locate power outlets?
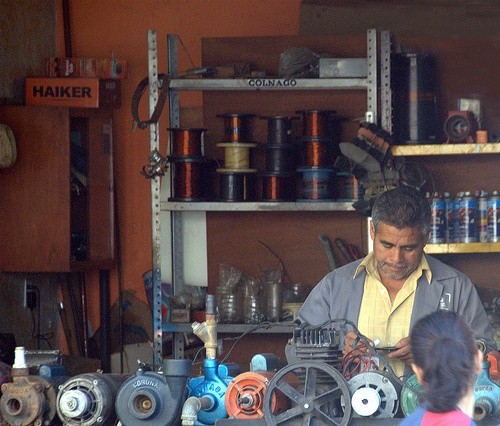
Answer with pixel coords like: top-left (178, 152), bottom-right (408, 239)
top-left (24, 279), bottom-right (37, 308)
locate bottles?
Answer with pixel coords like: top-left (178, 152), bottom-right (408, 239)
top-left (426, 191), bottom-right (500, 243)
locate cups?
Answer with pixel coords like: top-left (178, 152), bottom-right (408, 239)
top-left (260, 283), bottom-right (283, 322)
top-left (214, 285), bottom-right (242, 321)
top-left (242, 295), bottom-right (262, 323)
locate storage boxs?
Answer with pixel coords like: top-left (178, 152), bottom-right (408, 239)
top-left (25, 77), bottom-right (118, 109)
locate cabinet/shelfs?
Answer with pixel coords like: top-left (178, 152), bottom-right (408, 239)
top-left (149, 27), bottom-right (379, 371)
top-left (380, 30), bottom-right (500, 254)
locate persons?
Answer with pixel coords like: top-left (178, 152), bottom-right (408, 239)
top-left (285, 187), bottom-right (498, 418)
top-left (395, 310), bottom-right (483, 426)
